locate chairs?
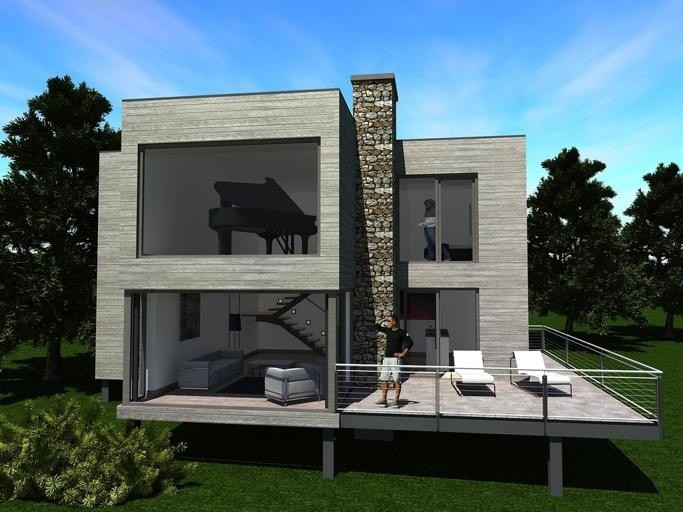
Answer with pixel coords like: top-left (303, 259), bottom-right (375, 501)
top-left (450, 348), bottom-right (498, 398)
top-left (507, 349), bottom-right (574, 397)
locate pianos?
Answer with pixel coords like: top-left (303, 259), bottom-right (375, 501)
top-left (207, 178), bottom-right (319, 253)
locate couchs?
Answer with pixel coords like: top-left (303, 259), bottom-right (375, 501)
top-left (264, 363), bottom-right (323, 407)
top-left (175, 350), bottom-right (247, 390)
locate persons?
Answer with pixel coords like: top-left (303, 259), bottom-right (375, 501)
top-left (415, 198), bottom-right (445, 262)
top-left (374, 315), bottom-right (414, 409)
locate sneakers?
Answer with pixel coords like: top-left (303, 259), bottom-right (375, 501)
top-left (392, 400), bottom-right (399, 408)
top-left (376, 400), bottom-right (387, 407)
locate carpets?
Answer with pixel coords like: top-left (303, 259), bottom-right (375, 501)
top-left (214, 376), bottom-right (268, 395)
